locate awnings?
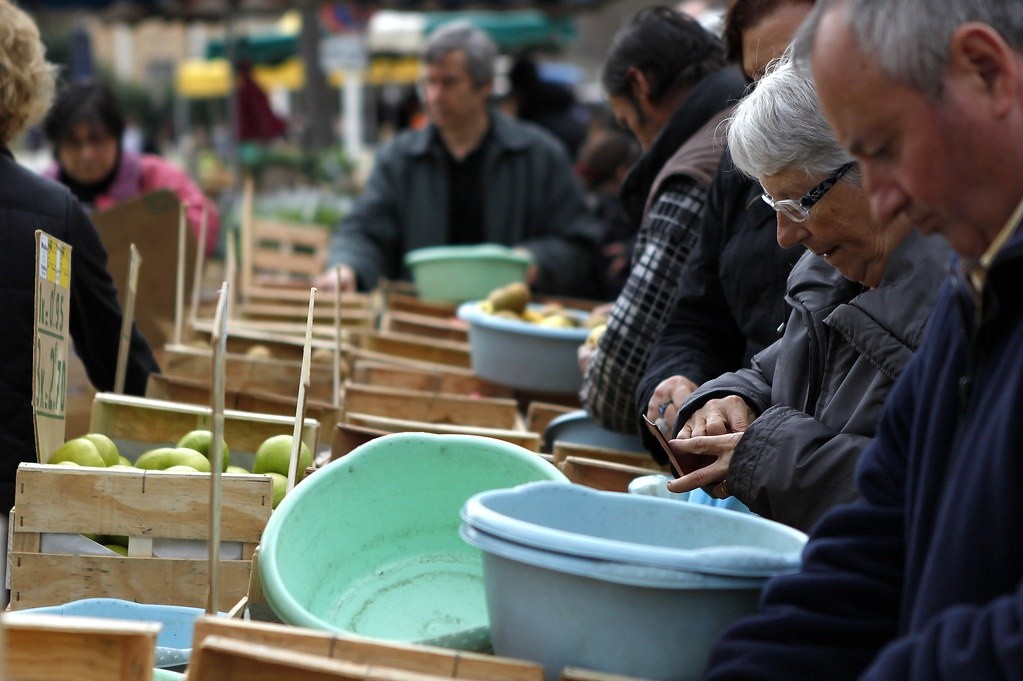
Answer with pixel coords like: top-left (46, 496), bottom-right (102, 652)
top-left (367, 11), bottom-right (575, 48)
top-left (207, 33), bottom-right (327, 63)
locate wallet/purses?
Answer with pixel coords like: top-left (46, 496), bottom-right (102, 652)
top-left (641, 411), bottom-right (740, 491)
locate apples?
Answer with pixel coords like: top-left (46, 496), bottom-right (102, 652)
top-left (48, 430), bottom-right (312, 557)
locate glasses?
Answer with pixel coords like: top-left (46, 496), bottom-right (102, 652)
top-left (762, 162), bottom-right (854, 222)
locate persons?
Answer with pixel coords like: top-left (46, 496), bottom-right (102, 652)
top-left (234, 60), bottom-right (290, 144)
top-left (316, 0), bottom-right (752, 303)
top-left (666, 60), bottom-right (954, 535)
top-left (39, 81), bottom-right (219, 253)
top-left (0, 0), bottom-right (161, 615)
top-left (633, 0), bottom-right (816, 470)
top-left (701, 0), bottom-right (1023, 681)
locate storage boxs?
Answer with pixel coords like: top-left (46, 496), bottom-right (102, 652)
top-left (0, 275), bottom-right (711, 681)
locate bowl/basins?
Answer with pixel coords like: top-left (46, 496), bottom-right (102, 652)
top-left (628, 474), bottom-right (764, 520)
top-left (258, 430), bottom-right (572, 657)
top-left (457, 482), bottom-right (815, 679)
top-left (541, 409), bottom-right (652, 454)
top-left (6, 598), bottom-right (231, 669)
top-left (456, 295), bottom-right (606, 394)
top-left (404, 243), bottom-right (532, 304)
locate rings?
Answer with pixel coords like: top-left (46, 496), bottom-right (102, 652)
top-left (721, 480), bottom-right (730, 497)
top-left (655, 418), bottom-right (670, 435)
top-left (659, 400), bottom-right (673, 418)
